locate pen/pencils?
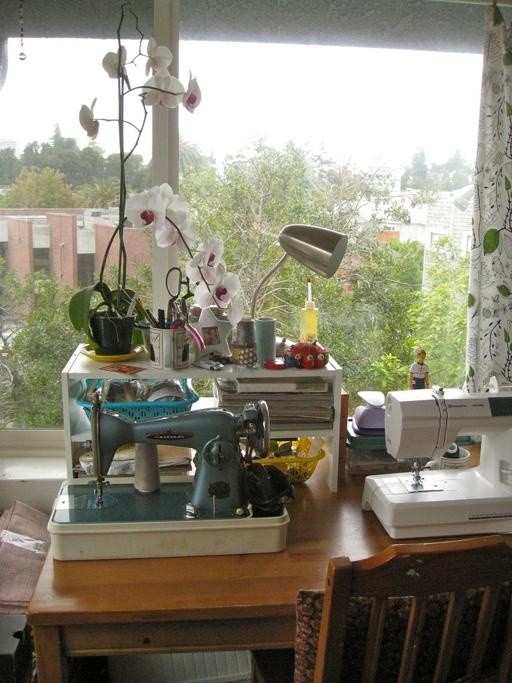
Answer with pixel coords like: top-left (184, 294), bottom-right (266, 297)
top-left (147, 309), bottom-right (165, 329)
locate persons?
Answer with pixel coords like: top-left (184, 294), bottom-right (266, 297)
top-left (408, 348), bottom-right (430, 389)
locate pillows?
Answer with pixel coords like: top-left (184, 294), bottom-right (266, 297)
top-left (293, 583), bottom-right (512, 683)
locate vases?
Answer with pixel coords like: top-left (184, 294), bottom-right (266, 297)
top-left (91, 313), bottom-right (134, 355)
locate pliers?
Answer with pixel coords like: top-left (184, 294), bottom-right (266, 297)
top-left (171, 299), bottom-right (205, 353)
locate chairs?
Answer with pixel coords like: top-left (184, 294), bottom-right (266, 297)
top-left (249, 533), bottom-right (512, 683)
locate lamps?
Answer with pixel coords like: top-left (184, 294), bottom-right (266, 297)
top-left (250, 223), bottom-right (349, 318)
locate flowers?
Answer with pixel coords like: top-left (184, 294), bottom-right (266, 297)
top-left (69, 2), bottom-right (246, 350)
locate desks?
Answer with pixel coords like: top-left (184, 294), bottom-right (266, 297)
top-left (27, 440), bottom-right (512, 683)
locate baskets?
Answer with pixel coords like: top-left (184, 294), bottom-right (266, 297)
top-left (75, 379), bottom-right (199, 421)
top-left (243, 441), bottom-right (326, 482)
